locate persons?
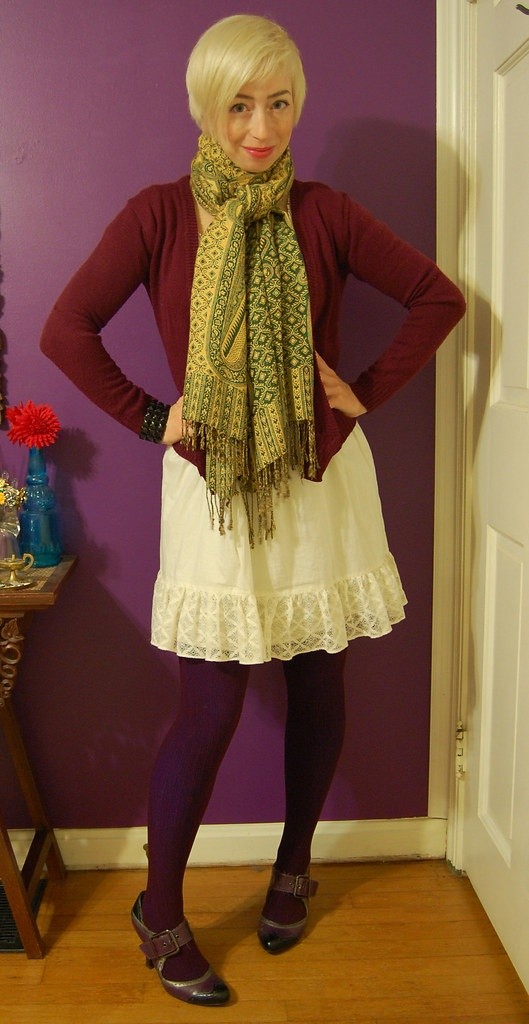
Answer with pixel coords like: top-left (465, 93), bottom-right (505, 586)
top-left (39, 13), bottom-right (470, 1006)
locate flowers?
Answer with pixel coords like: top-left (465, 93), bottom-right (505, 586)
top-left (4, 400), bottom-right (63, 449)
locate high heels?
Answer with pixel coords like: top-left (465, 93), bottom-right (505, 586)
top-left (258, 861), bottom-right (319, 955)
top-left (131, 890), bottom-right (230, 1005)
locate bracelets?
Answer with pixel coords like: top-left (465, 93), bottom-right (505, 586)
top-left (140, 397), bottom-right (169, 444)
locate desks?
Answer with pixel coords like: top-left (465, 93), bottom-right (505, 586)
top-left (0, 553), bottom-right (80, 958)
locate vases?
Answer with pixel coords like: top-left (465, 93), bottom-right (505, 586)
top-left (18, 447), bottom-right (64, 569)
